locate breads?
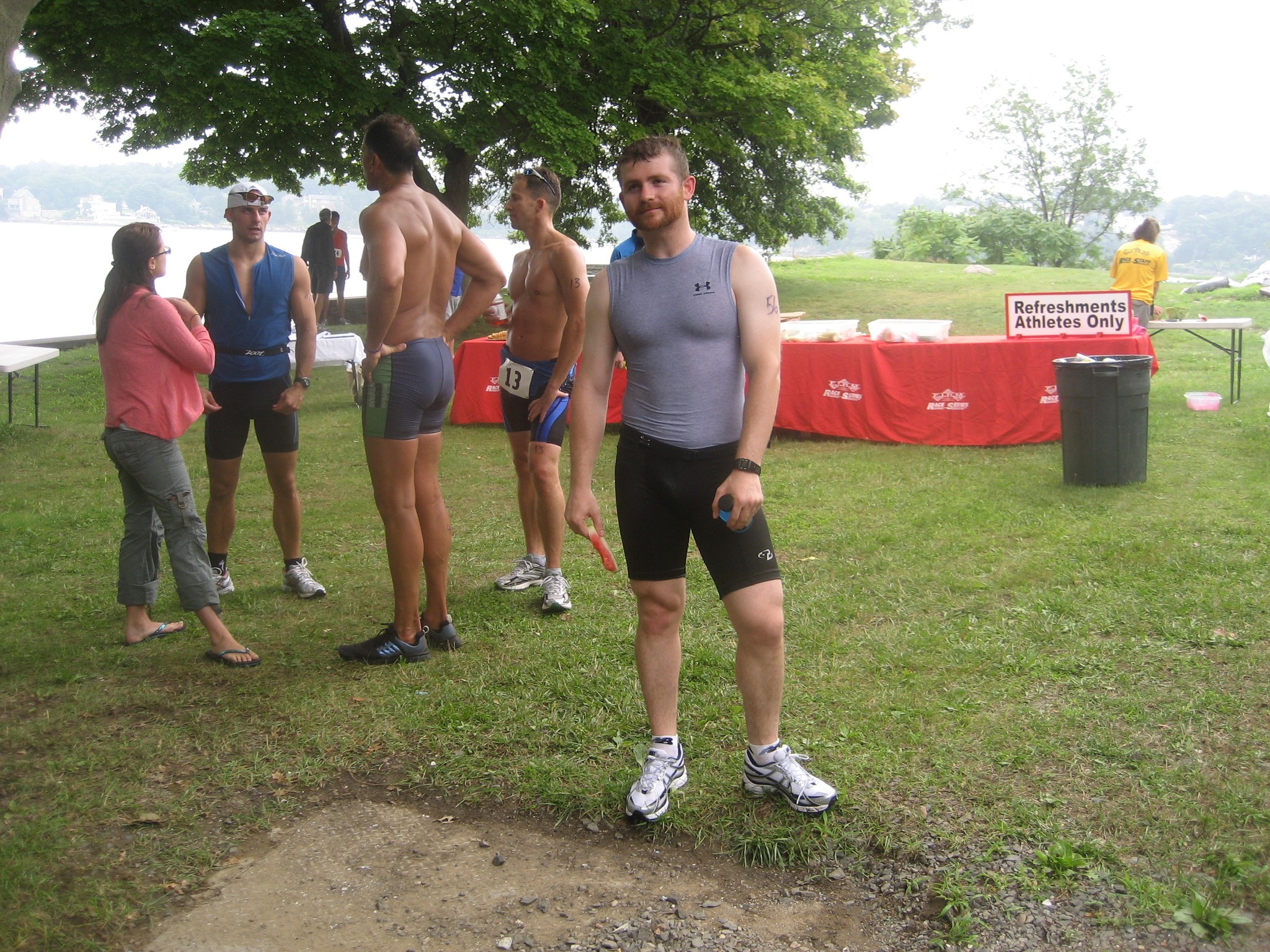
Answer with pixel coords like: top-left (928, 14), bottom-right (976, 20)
top-left (487, 330), bottom-right (507, 339)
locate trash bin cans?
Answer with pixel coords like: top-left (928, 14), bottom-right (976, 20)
top-left (1051, 354), bottom-right (1152, 487)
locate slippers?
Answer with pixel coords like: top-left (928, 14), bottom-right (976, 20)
top-left (125, 622), bottom-right (186, 646)
top-left (205, 647), bottom-right (261, 668)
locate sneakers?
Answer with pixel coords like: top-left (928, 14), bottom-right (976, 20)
top-left (211, 567), bottom-right (235, 595)
top-left (338, 621), bottom-right (430, 665)
top-left (281, 557), bottom-right (326, 598)
top-left (539, 573), bottom-right (572, 611)
top-left (742, 743), bottom-right (838, 812)
top-left (419, 611), bottom-right (462, 651)
top-left (626, 742), bottom-right (688, 821)
top-left (496, 554), bottom-right (546, 590)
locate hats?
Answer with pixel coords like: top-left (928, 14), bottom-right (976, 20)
top-left (227, 182), bottom-right (270, 208)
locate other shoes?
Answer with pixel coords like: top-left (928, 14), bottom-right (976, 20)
top-left (316, 323), bottom-right (329, 332)
top-left (337, 317), bottom-right (351, 325)
top-left (320, 318), bottom-right (329, 326)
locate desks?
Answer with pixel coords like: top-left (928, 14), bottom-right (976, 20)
top-left (0, 343), bottom-right (60, 430)
top-left (451, 327), bottom-right (1151, 448)
top-left (286, 333), bottom-right (361, 410)
top-left (1151, 317), bottom-right (1252, 404)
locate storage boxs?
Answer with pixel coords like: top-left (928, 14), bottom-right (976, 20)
top-left (781, 319), bottom-right (860, 343)
top-left (867, 319), bottom-right (952, 342)
top-left (1183, 391), bottom-right (1222, 411)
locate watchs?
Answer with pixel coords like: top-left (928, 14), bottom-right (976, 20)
top-left (294, 377), bottom-right (311, 387)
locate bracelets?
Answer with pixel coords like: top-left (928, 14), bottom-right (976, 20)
top-left (734, 458), bottom-right (761, 476)
top-left (364, 342), bottom-right (383, 354)
top-left (190, 314), bottom-right (200, 321)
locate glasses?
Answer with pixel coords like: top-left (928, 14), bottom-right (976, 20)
top-left (145, 246), bottom-right (171, 264)
top-left (228, 192), bottom-right (275, 205)
top-left (523, 167), bottom-right (557, 196)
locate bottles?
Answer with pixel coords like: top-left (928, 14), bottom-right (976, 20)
top-left (718, 495), bottom-right (754, 533)
top-left (487, 293), bottom-right (509, 326)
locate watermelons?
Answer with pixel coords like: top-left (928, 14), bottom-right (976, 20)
top-left (1166, 306), bottom-right (1192, 321)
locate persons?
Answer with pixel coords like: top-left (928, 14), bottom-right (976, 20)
top-left (182, 182), bottom-right (326, 599)
top-left (609, 228), bottom-right (647, 369)
top-left (96, 222), bottom-right (258, 667)
top-left (1111, 218), bottom-right (1168, 332)
top-left (483, 166), bottom-right (590, 613)
top-left (446, 266), bottom-right (465, 361)
top-left (337, 114), bottom-right (506, 665)
top-left (301, 208), bottom-right (350, 333)
top-left (564, 135), bottom-right (839, 825)
